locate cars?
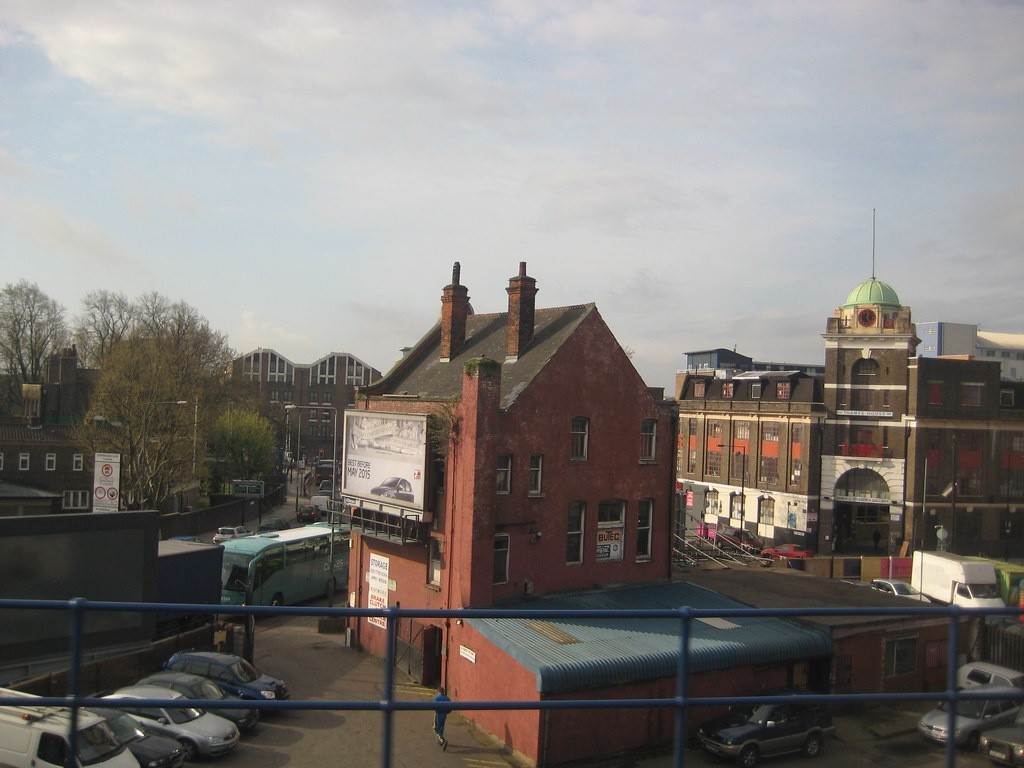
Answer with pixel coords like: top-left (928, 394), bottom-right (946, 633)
top-left (80, 705), bottom-right (186, 768)
top-left (917, 684), bottom-right (1024, 752)
top-left (372, 477), bottom-right (414, 504)
top-left (978, 703), bottom-right (1024, 768)
top-left (98, 684), bottom-right (240, 763)
top-left (135, 670), bottom-right (260, 735)
top-left (695, 687), bottom-right (835, 768)
top-left (697, 523), bottom-right (763, 552)
top-left (259, 516), bottom-right (291, 534)
top-left (872, 578), bottom-right (933, 605)
top-left (760, 543), bottom-right (814, 567)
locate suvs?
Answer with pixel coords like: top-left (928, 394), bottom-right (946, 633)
top-left (212, 524), bottom-right (253, 545)
top-left (162, 649), bottom-right (290, 717)
top-left (297, 503), bottom-right (321, 523)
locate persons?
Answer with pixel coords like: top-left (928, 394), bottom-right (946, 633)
top-left (874, 528), bottom-right (881, 549)
top-left (431, 687), bottom-right (452, 751)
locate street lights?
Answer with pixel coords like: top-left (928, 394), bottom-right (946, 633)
top-left (140, 400), bottom-right (188, 511)
top-left (271, 400), bottom-right (293, 482)
top-left (285, 405), bottom-right (350, 617)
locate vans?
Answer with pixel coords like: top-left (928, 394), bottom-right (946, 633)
top-left (0, 684), bottom-right (141, 768)
top-left (957, 662), bottom-right (1024, 693)
top-left (311, 458), bottom-right (340, 517)
top-left (911, 550), bottom-right (1024, 626)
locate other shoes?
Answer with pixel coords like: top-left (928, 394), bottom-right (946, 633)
top-left (442, 740), bottom-right (448, 751)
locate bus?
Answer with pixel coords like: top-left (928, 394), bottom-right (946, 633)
top-left (218, 523), bottom-right (351, 608)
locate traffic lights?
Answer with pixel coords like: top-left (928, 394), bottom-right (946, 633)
top-left (319, 448), bottom-right (325, 458)
